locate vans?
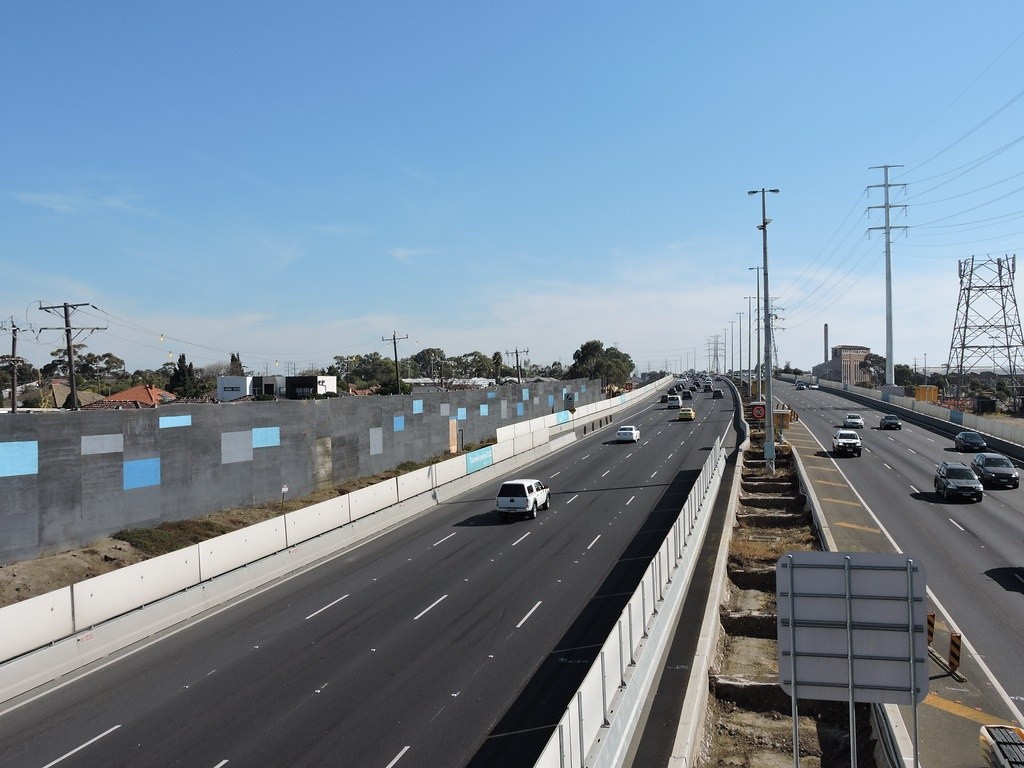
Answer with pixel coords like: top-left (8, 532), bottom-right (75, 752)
top-left (667, 396), bottom-right (683, 409)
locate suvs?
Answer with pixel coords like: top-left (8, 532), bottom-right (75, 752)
top-left (933, 461), bottom-right (983, 503)
top-left (496, 479), bottom-right (550, 519)
top-left (832, 430), bottom-right (863, 457)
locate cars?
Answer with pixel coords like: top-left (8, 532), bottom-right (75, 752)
top-left (880, 414), bottom-right (902, 430)
top-left (713, 389), bottom-right (724, 399)
top-left (615, 425), bottom-right (641, 443)
top-left (677, 407), bottom-right (696, 420)
top-left (795, 380), bottom-right (818, 390)
top-left (843, 414), bottom-right (864, 428)
top-left (970, 454), bottom-right (1020, 489)
top-left (660, 394), bottom-right (671, 403)
top-left (954, 432), bottom-right (989, 453)
top-left (667, 369), bottom-right (722, 399)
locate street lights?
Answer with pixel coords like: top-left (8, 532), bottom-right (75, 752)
top-left (749, 187), bottom-right (782, 477)
top-left (721, 266), bottom-right (764, 402)
